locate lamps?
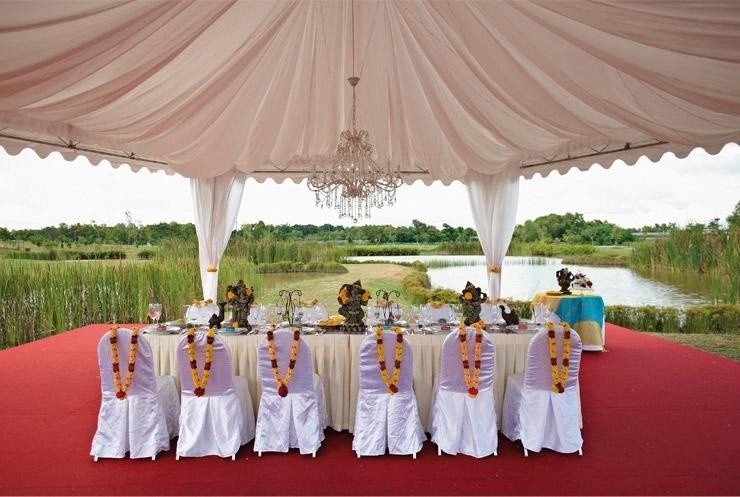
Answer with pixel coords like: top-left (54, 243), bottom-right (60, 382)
top-left (307, 0), bottom-right (405, 223)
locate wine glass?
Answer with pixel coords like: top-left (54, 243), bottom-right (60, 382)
top-left (148, 298), bottom-right (552, 335)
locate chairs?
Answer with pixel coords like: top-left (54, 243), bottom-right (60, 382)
top-left (253, 327), bottom-right (329, 457)
top-left (351, 324), bottom-right (428, 458)
top-left (176, 328), bottom-right (255, 461)
top-left (501, 321), bottom-right (583, 457)
top-left (89, 326), bottom-right (181, 462)
top-left (427, 323), bottom-right (499, 458)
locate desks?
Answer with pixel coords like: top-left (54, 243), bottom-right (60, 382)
top-left (532, 291), bottom-right (605, 351)
top-left (137, 322), bottom-right (583, 433)
